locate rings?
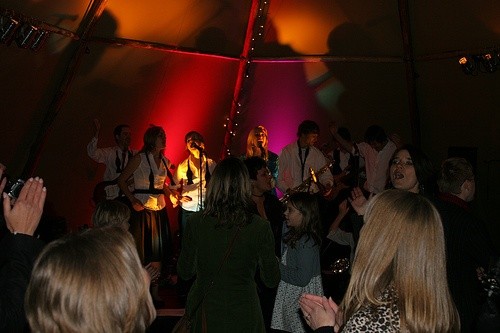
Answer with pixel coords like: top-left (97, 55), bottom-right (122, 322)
top-left (306, 314), bottom-right (311, 320)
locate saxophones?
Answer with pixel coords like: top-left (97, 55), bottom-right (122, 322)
top-left (280, 159), bottom-right (338, 203)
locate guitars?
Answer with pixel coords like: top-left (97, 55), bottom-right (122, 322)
top-left (93, 179), bottom-right (180, 210)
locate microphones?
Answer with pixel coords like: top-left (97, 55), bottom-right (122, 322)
top-left (257, 143), bottom-right (266, 152)
top-left (192, 143), bottom-right (203, 152)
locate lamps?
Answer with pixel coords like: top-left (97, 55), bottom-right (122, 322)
top-left (456, 49), bottom-right (500, 74)
top-left (0, 15), bottom-right (49, 49)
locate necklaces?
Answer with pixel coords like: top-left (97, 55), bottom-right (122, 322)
top-left (252, 193), bottom-right (265, 197)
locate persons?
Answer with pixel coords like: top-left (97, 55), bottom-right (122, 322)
top-left (87, 118), bottom-right (139, 201)
top-left (166, 131), bottom-right (217, 306)
top-left (172, 157), bottom-right (281, 333)
top-left (23, 224), bottom-right (157, 333)
top-left (117, 126), bottom-right (192, 307)
top-left (239, 119), bottom-right (500, 333)
top-left (299, 189), bottom-right (461, 333)
top-left (0, 163), bottom-right (47, 333)
top-left (92, 199), bottom-right (161, 289)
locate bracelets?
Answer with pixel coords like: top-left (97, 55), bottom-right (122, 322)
top-left (11, 232), bottom-right (34, 240)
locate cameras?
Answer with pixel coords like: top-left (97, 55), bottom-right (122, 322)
top-left (3, 173), bottom-right (26, 209)
